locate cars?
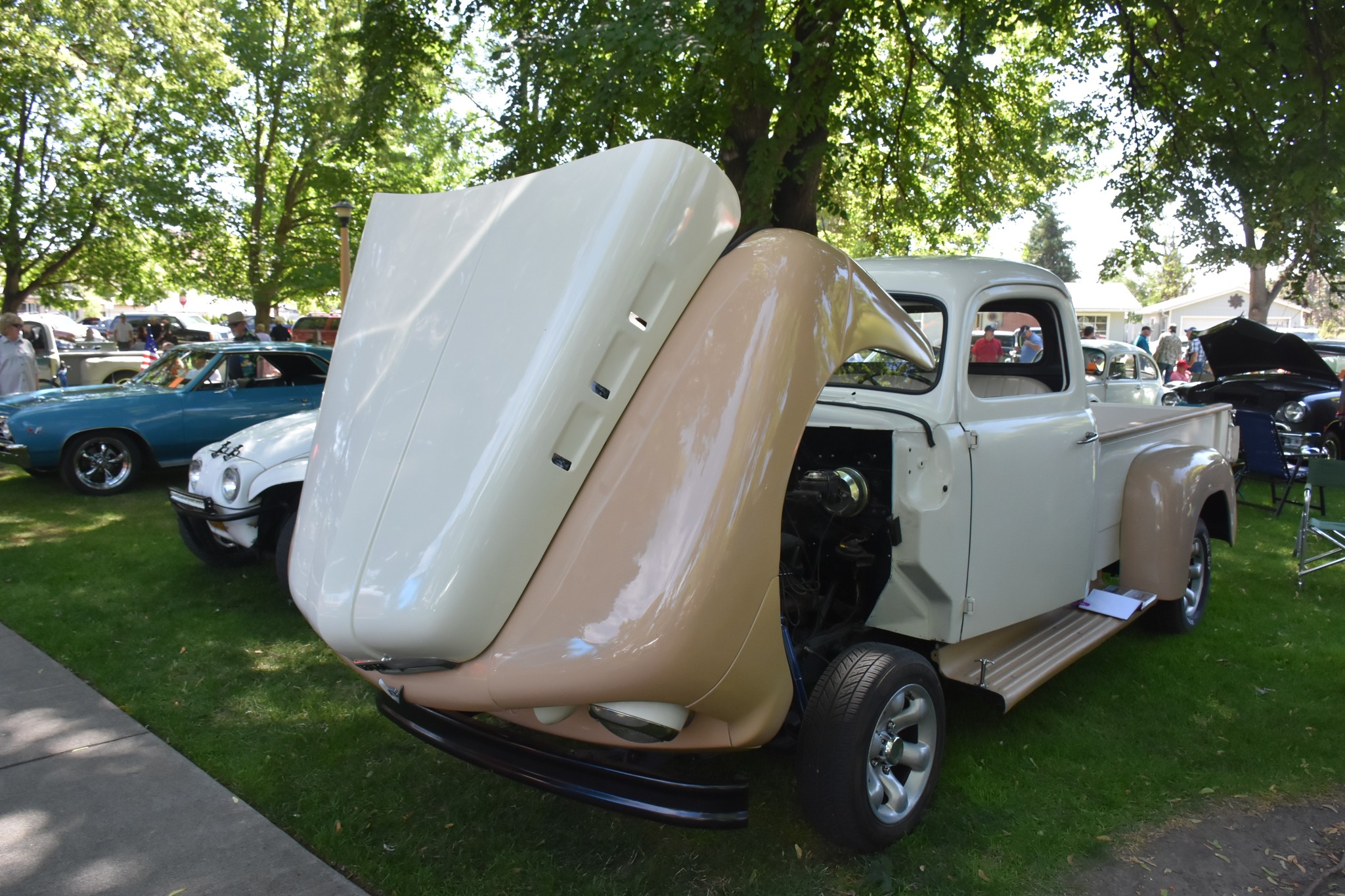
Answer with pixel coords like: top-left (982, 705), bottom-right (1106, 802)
top-left (970, 327), bottom-right (1043, 357)
top-left (1031, 339), bottom-right (1171, 408)
top-left (168, 408), bottom-right (322, 601)
top-left (1161, 314), bottom-right (1345, 483)
top-left (77, 317), bottom-right (113, 339)
top-left (845, 346), bottom-right (941, 363)
top-left (219, 315), bottom-right (292, 336)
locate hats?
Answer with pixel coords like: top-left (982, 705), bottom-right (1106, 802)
top-left (1142, 326), bottom-right (1154, 332)
top-left (1019, 325), bottom-right (1029, 334)
top-left (985, 325), bottom-right (996, 331)
top-left (1177, 359), bottom-right (1191, 369)
top-left (1184, 327), bottom-right (1196, 332)
top-left (226, 312), bottom-right (253, 324)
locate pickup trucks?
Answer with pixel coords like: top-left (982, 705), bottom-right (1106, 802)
top-left (16, 312), bottom-right (164, 390)
top-left (286, 138), bottom-right (1240, 856)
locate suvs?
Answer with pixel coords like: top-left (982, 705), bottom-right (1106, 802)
top-left (291, 310), bottom-right (341, 347)
top-left (106, 311), bottom-right (235, 344)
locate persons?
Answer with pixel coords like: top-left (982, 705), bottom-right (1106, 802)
top-left (161, 342), bottom-right (185, 375)
top-left (269, 316), bottom-right (292, 342)
top-left (0, 313), bottom-right (40, 394)
top-left (1169, 360), bottom-right (1191, 382)
top-left (1152, 323), bottom-right (1182, 385)
top-left (1013, 325), bottom-right (1044, 363)
top-left (970, 325), bottom-right (1004, 363)
top-left (131, 316), bottom-right (178, 351)
top-left (113, 312), bottom-right (134, 351)
top-left (1081, 326), bottom-right (1095, 340)
top-left (1184, 327), bottom-right (1207, 373)
top-left (1134, 326), bottom-right (1154, 370)
top-left (1078, 323), bottom-right (1097, 340)
top-left (226, 312), bottom-right (261, 380)
top-left (254, 324), bottom-right (272, 341)
top-left (184, 351), bottom-right (222, 384)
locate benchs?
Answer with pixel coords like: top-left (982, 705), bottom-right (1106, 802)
top-left (826, 373), bottom-right (1052, 398)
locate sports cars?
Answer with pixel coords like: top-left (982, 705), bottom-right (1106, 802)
top-left (0, 340), bottom-right (333, 495)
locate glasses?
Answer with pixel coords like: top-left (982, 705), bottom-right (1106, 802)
top-left (12, 323), bottom-right (23, 329)
top-left (226, 323), bottom-right (239, 328)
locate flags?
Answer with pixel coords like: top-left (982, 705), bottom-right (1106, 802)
top-left (140, 328), bottom-right (160, 372)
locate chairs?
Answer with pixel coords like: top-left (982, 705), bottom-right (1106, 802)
top-left (1191, 362), bottom-right (1205, 382)
top-left (1232, 410), bottom-right (1327, 520)
top-left (1293, 458), bottom-right (1345, 590)
